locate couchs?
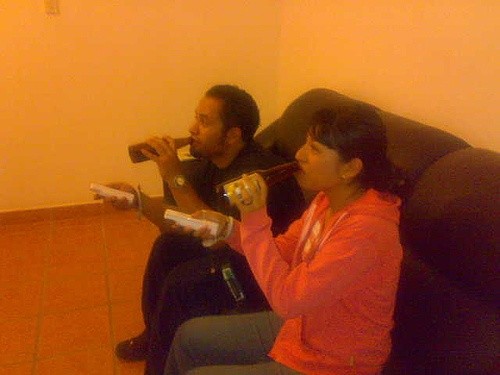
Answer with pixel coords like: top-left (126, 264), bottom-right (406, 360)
top-left (163, 88), bottom-right (500, 375)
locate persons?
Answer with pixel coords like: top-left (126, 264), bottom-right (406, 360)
top-left (161, 103), bottom-right (411, 374)
top-left (93, 84), bottom-right (308, 374)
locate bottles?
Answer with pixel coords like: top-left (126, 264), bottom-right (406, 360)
top-left (211, 161), bottom-right (301, 215)
top-left (128, 137), bottom-right (195, 163)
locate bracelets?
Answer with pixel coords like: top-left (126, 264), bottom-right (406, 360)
top-left (164, 170), bottom-right (192, 190)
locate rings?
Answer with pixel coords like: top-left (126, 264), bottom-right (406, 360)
top-left (241, 199), bottom-right (253, 205)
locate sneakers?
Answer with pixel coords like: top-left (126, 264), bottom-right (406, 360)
top-left (115, 332), bottom-right (152, 360)
top-left (144, 351), bottom-right (166, 375)
